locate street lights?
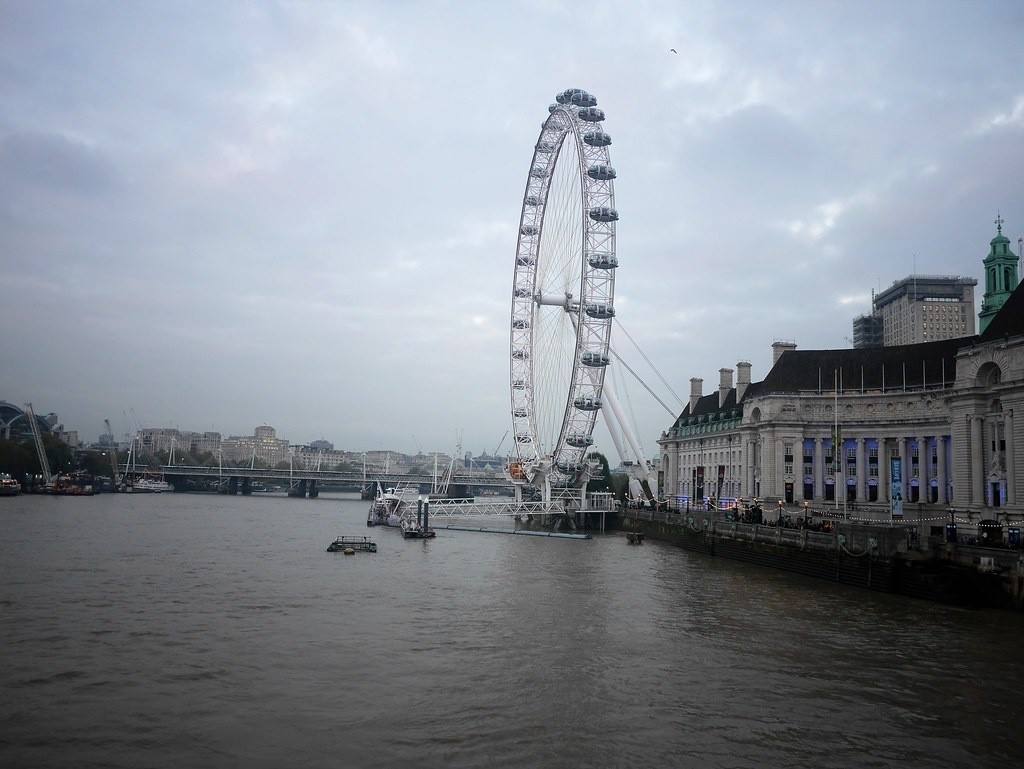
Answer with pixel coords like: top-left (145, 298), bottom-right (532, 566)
top-left (777, 499), bottom-right (784, 526)
top-left (735, 498), bottom-right (738, 518)
top-left (950, 505), bottom-right (956, 543)
top-left (638, 493), bottom-right (641, 502)
top-left (805, 501), bottom-right (809, 528)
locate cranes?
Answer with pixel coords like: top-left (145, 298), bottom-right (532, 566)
top-left (102, 418), bottom-right (119, 475)
top-left (24, 400), bottom-right (52, 481)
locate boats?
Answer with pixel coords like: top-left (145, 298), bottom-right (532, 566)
top-left (327, 534), bottom-right (377, 553)
top-left (0, 466), bottom-right (175, 496)
top-left (186, 478), bottom-right (284, 494)
